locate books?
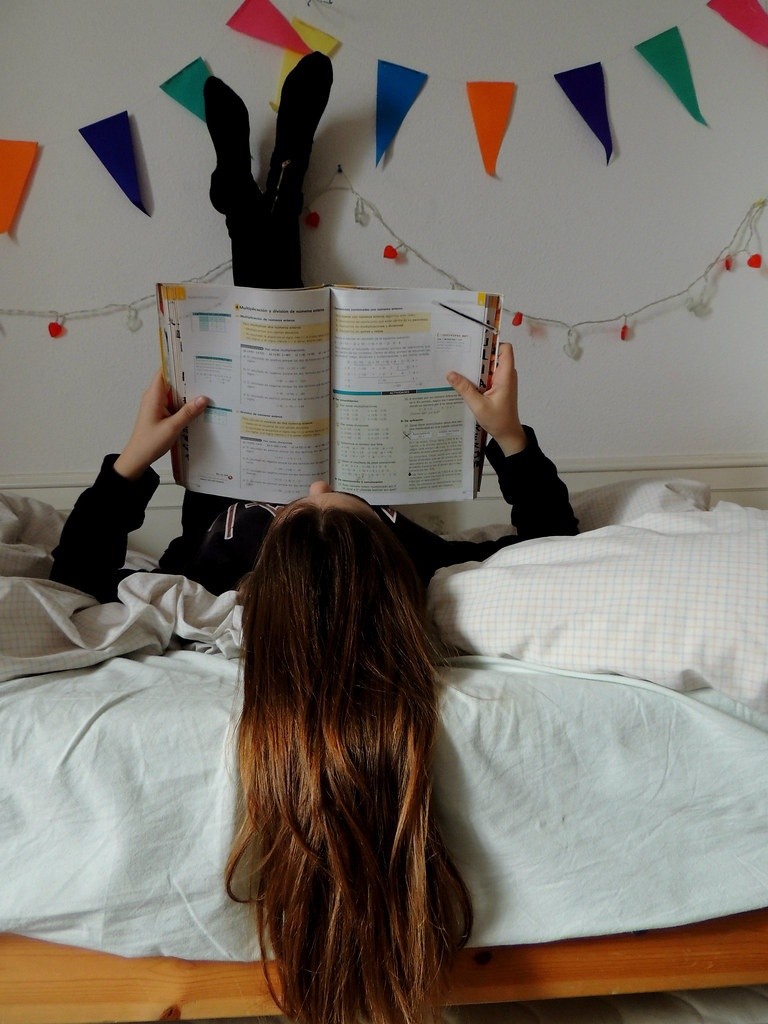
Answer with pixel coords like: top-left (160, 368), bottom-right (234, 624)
top-left (153, 282), bottom-right (503, 509)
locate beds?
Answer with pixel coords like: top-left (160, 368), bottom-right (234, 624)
top-left (0, 472), bottom-right (768, 1024)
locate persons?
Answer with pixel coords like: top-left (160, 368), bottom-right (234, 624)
top-left (47, 49), bottom-right (586, 1021)
